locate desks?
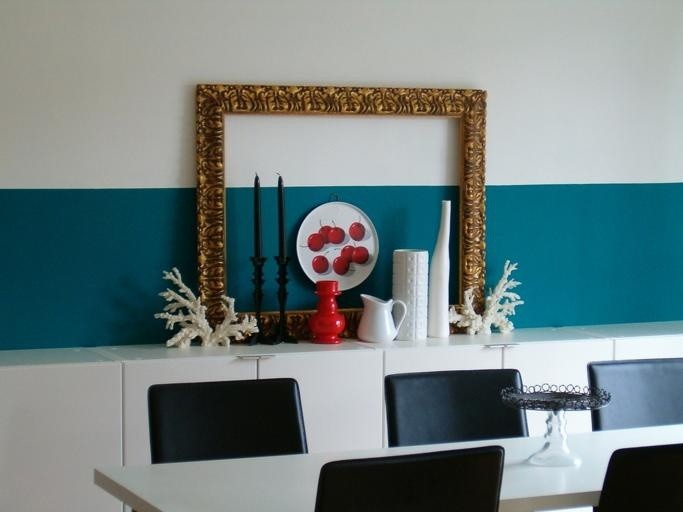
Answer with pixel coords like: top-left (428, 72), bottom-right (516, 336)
top-left (95, 425), bottom-right (683, 512)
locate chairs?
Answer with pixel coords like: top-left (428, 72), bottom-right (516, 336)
top-left (147, 378), bottom-right (308, 463)
top-left (385, 369), bottom-right (530, 446)
top-left (596, 444), bottom-right (683, 512)
top-left (315, 447), bottom-right (504, 512)
top-left (588, 357), bottom-right (683, 431)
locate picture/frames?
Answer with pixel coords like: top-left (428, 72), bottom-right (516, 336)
top-left (196, 84), bottom-right (487, 343)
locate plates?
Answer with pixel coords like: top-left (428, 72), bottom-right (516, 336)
top-left (296, 202), bottom-right (379, 291)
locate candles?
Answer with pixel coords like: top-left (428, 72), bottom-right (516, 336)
top-left (278, 175), bottom-right (286, 257)
top-left (254, 176), bottom-right (261, 258)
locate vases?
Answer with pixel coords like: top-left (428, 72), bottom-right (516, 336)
top-left (428, 200), bottom-right (450, 338)
top-left (392, 248), bottom-right (429, 341)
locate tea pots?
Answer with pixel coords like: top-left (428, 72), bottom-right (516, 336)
top-left (357, 293), bottom-right (407, 343)
